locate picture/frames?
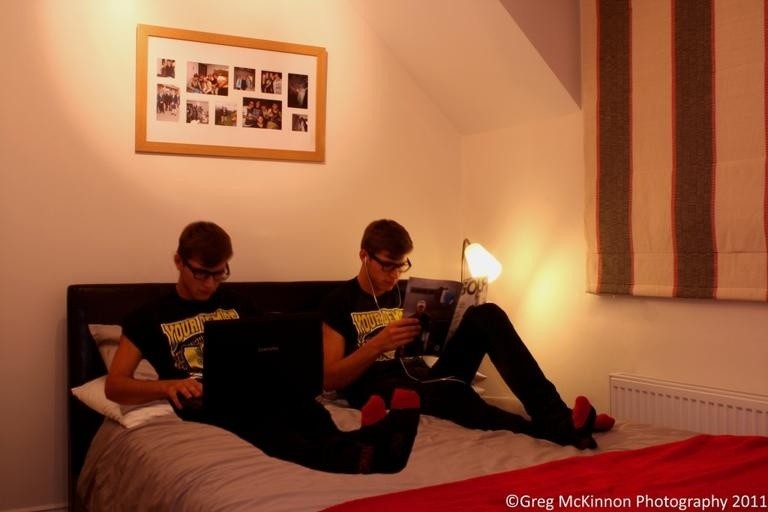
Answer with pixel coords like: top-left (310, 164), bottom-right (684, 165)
top-left (133, 22), bottom-right (329, 166)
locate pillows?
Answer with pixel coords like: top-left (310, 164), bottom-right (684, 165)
top-left (87, 316), bottom-right (170, 417)
top-left (401, 355), bottom-right (488, 385)
top-left (70, 372), bottom-right (174, 430)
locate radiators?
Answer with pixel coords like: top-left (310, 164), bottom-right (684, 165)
top-left (608, 368), bottom-right (768, 438)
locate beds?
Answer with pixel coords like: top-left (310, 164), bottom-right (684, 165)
top-left (66, 281), bottom-right (768, 512)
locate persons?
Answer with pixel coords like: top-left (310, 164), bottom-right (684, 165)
top-left (158, 59), bottom-right (282, 128)
top-left (104, 222), bottom-right (420, 474)
top-left (321, 220), bottom-right (616, 450)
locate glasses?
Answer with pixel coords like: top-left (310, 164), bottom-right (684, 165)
top-left (371, 257), bottom-right (412, 273)
top-left (186, 262), bottom-right (230, 281)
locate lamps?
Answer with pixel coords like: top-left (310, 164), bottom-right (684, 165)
top-left (460, 237), bottom-right (505, 286)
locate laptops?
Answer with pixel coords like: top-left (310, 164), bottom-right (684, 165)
top-left (178, 310), bottom-right (324, 423)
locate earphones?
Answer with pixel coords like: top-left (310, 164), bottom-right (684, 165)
top-left (362, 255), bottom-right (367, 264)
